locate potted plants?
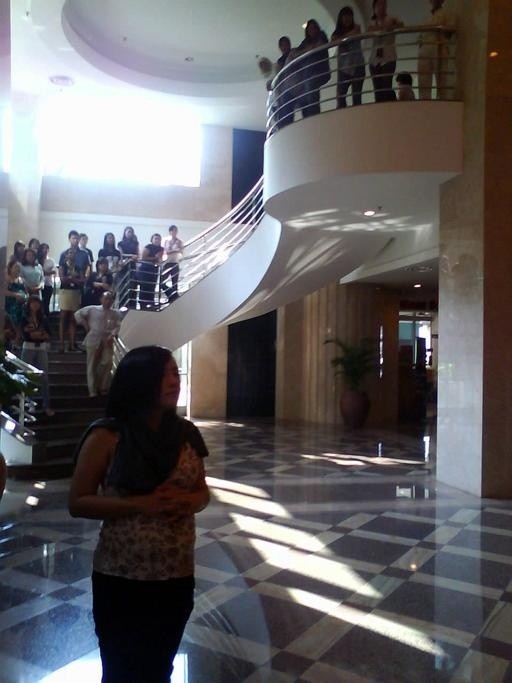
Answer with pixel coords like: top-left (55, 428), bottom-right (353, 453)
top-left (323, 337), bottom-right (382, 426)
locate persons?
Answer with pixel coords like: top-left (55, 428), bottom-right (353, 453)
top-left (4, 222), bottom-right (184, 354)
top-left (19, 295), bottom-right (56, 417)
top-left (73, 290), bottom-right (123, 397)
top-left (66, 343), bottom-right (212, 683)
top-left (257, 0), bottom-right (458, 130)
